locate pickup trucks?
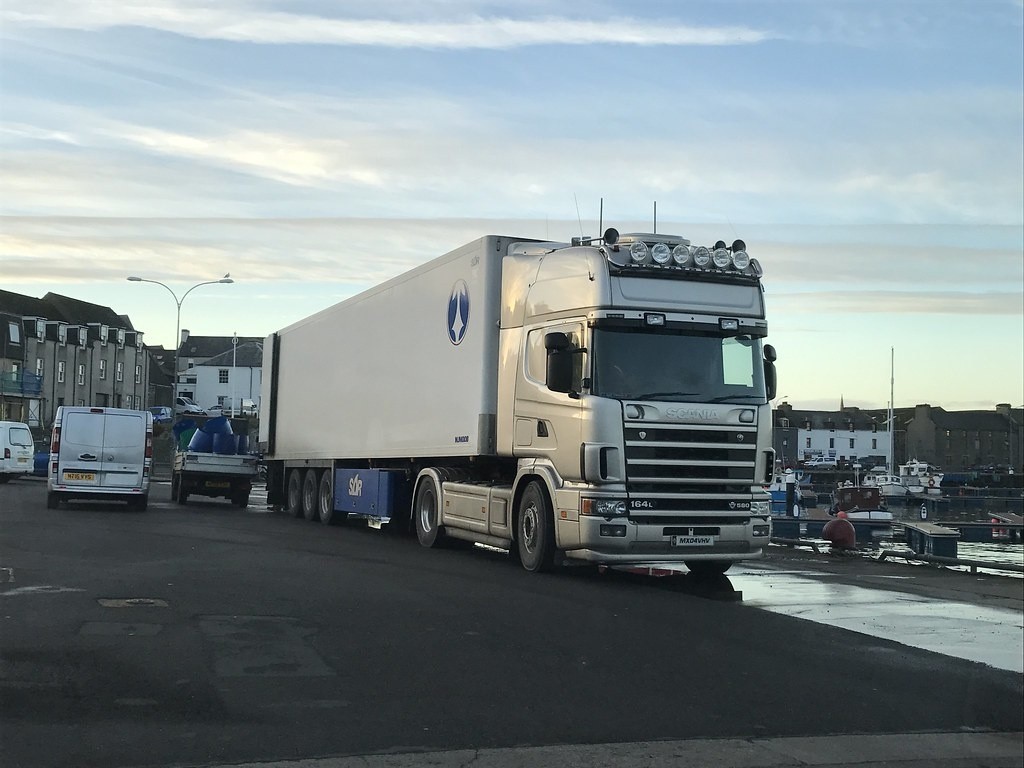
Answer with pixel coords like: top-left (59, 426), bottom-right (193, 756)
top-left (845, 456), bottom-right (878, 470)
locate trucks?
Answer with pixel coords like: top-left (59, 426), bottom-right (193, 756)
top-left (171, 413), bottom-right (258, 512)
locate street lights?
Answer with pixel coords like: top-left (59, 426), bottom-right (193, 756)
top-left (127, 276), bottom-right (235, 450)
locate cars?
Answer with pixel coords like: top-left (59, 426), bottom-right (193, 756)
top-left (33, 440), bottom-right (49, 478)
top-left (202, 405), bottom-right (224, 417)
top-left (146, 406), bottom-right (173, 425)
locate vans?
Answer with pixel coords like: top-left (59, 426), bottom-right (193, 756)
top-left (220, 397), bottom-right (259, 418)
top-left (176, 397), bottom-right (204, 415)
top-left (0, 421), bottom-right (34, 484)
top-left (803, 456), bottom-right (837, 470)
top-left (44, 405), bottom-right (154, 512)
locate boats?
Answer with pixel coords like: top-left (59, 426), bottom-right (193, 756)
top-left (863, 464), bottom-right (909, 496)
top-left (898, 458), bottom-right (946, 494)
top-left (767, 471), bottom-right (818, 515)
top-left (823, 464), bottom-right (892, 524)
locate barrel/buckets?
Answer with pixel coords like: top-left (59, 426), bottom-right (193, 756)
top-left (172, 416), bottom-right (250, 455)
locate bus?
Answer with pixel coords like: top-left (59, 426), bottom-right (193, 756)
top-left (803, 448), bottom-right (837, 460)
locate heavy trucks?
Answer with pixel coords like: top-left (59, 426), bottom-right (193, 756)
top-left (257, 199), bottom-right (776, 576)
